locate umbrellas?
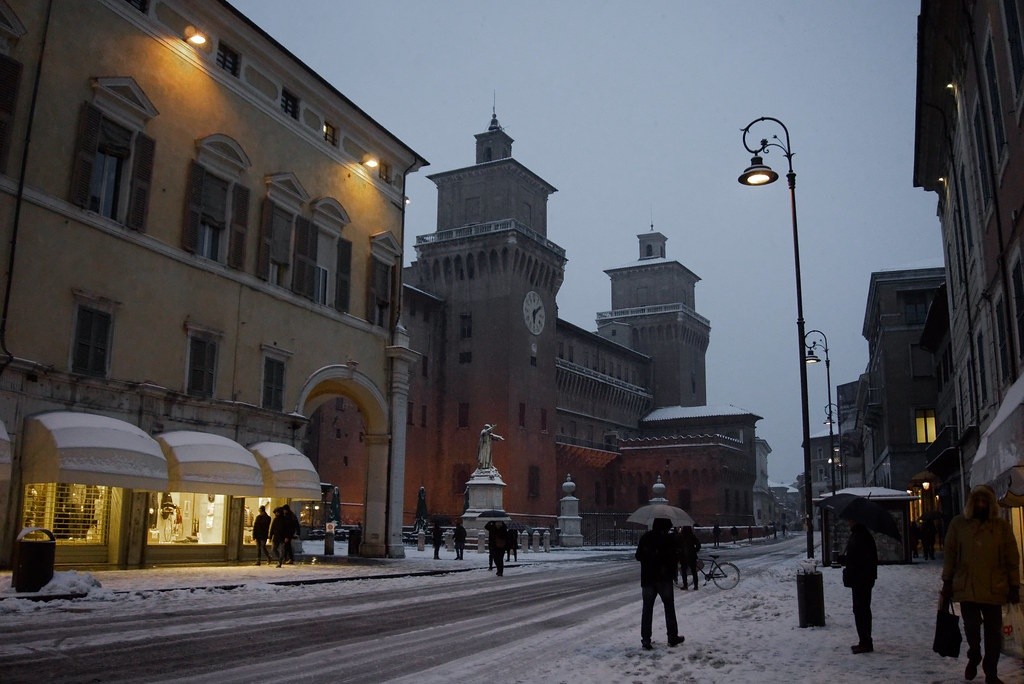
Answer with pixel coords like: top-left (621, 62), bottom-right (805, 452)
top-left (626, 500), bottom-right (695, 528)
top-left (815, 493), bottom-right (903, 555)
top-left (477, 510), bottom-right (511, 521)
top-left (506, 520), bottom-right (525, 529)
top-left (414, 487), bottom-right (428, 533)
top-left (920, 511), bottom-right (943, 520)
top-left (463, 486), bottom-right (469, 513)
top-left (327, 489), bottom-right (341, 522)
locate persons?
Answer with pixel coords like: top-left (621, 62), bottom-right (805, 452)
top-left (940, 484), bottom-right (1021, 684)
top-left (252, 506), bottom-right (272, 565)
top-left (910, 519), bottom-right (937, 560)
top-left (713, 524), bottom-right (721, 547)
top-left (748, 525), bottom-right (753, 541)
top-left (671, 526), bottom-right (701, 591)
top-left (270, 505), bottom-right (300, 567)
top-left (781, 524), bottom-right (786, 534)
top-left (836, 518), bottom-right (878, 654)
top-left (477, 423), bottom-right (505, 469)
top-left (731, 525), bottom-right (738, 544)
top-left (432, 522), bottom-right (443, 559)
top-left (484, 520), bottom-right (519, 577)
top-left (772, 525), bottom-right (778, 539)
top-left (636, 518), bottom-right (684, 649)
top-left (452, 523), bottom-right (467, 560)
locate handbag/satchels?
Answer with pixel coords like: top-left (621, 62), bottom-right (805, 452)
top-left (933, 596), bottom-right (963, 659)
top-left (843, 568), bottom-right (852, 588)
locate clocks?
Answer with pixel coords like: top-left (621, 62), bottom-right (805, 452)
top-left (523, 291), bottom-right (545, 335)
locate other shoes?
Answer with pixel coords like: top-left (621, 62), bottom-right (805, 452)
top-left (455, 557), bottom-right (460, 560)
top-left (850, 645), bottom-right (874, 654)
top-left (286, 559), bottom-right (293, 564)
top-left (668, 636), bottom-right (684, 647)
top-left (985, 676), bottom-right (1004, 684)
top-left (680, 587), bottom-right (688, 590)
top-left (254, 562), bottom-right (261, 566)
top-left (642, 644), bottom-right (653, 650)
top-left (460, 558), bottom-right (463, 560)
top-left (505, 560), bottom-right (510, 562)
top-left (489, 567), bottom-right (492, 570)
top-left (276, 565), bottom-right (281, 568)
top-left (267, 555), bottom-right (272, 565)
top-left (965, 659), bottom-right (981, 681)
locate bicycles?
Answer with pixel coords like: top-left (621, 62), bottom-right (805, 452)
top-left (673, 555), bottom-right (742, 590)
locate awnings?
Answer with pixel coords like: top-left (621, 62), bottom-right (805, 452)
top-left (969, 372), bottom-right (1024, 503)
top-left (0, 408), bottom-right (323, 502)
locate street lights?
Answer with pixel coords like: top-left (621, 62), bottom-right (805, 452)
top-left (823, 402), bottom-right (845, 490)
top-left (803, 328), bottom-right (842, 567)
top-left (737, 116), bottom-right (826, 628)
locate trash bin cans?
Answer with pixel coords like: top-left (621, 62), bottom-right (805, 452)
top-left (348, 529), bottom-right (361, 555)
top-left (11, 527), bottom-right (56, 594)
